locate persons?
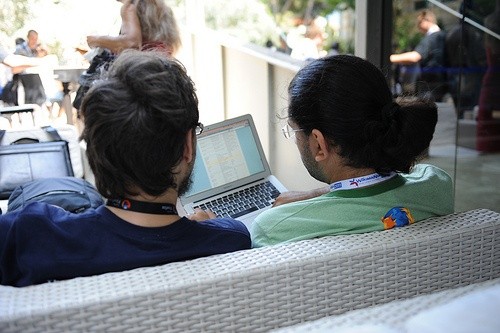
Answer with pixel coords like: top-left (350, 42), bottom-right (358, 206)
top-left (249, 55), bottom-right (454, 247)
top-left (0, 46), bottom-right (252, 286)
top-left (10, 31), bottom-right (48, 76)
top-left (87, 0), bottom-right (180, 66)
top-left (390, 10), bottom-right (450, 98)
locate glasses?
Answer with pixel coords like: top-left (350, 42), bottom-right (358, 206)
top-left (281, 124), bottom-right (330, 140)
top-left (187, 123), bottom-right (204, 139)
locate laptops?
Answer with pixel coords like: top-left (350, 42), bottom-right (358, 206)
top-left (176, 114), bottom-right (288, 223)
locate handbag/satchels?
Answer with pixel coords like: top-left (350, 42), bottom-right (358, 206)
top-left (70, 50), bottom-right (111, 112)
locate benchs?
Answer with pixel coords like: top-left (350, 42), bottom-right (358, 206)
top-left (0, 207), bottom-right (499, 332)
